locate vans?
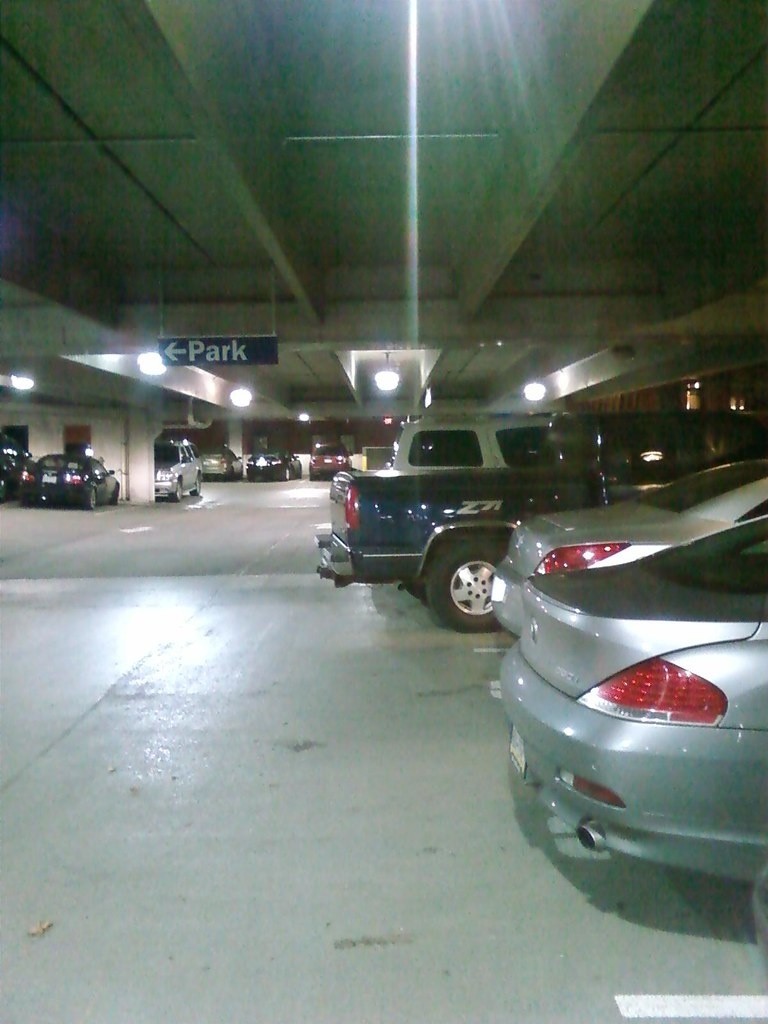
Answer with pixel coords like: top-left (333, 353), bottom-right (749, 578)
top-left (153, 440), bottom-right (202, 501)
top-left (393, 417), bottom-right (553, 466)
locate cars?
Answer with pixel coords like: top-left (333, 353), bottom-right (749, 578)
top-left (245, 450), bottom-right (302, 481)
top-left (23, 453), bottom-right (120, 510)
top-left (200, 447), bottom-right (243, 481)
top-left (500, 515), bottom-right (768, 896)
top-left (493, 458), bottom-right (768, 634)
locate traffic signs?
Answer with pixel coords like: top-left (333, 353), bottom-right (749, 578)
top-left (157, 333), bottom-right (278, 365)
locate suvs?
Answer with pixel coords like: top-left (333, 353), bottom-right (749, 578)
top-left (1, 434), bottom-right (32, 502)
top-left (310, 441), bottom-right (353, 479)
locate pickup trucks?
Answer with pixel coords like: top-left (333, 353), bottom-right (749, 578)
top-left (315, 409), bottom-right (767, 632)
